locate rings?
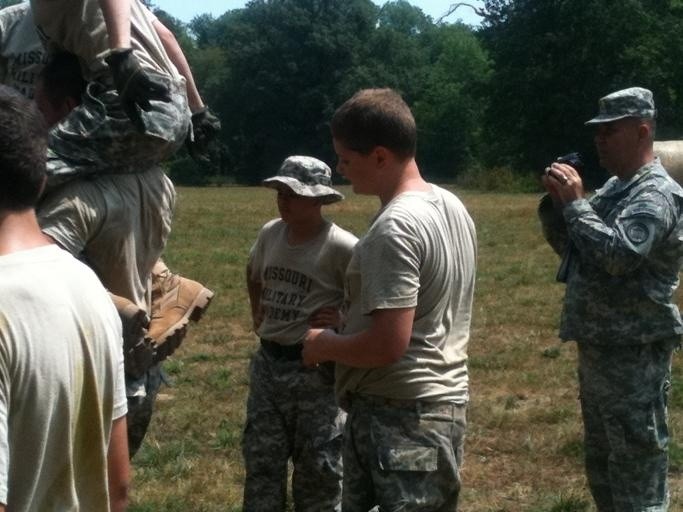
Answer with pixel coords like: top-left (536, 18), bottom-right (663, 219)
top-left (561, 177), bottom-right (567, 184)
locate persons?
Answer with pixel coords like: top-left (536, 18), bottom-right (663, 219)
top-left (241, 156), bottom-right (359, 512)
top-left (537, 87), bottom-right (683, 512)
top-left (302, 88), bottom-right (477, 512)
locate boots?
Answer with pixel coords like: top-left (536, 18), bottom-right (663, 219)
top-left (107, 257), bottom-right (215, 380)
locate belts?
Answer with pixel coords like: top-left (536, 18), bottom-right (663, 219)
top-left (260, 337), bottom-right (302, 358)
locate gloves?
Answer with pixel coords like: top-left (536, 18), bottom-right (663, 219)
top-left (103, 47), bottom-right (173, 135)
top-left (190, 106), bottom-right (222, 144)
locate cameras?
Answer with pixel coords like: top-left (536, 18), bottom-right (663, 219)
top-left (547, 151), bottom-right (586, 180)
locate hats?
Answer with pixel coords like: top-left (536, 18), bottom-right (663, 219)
top-left (583, 86), bottom-right (658, 128)
top-left (263, 155), bottom-right (344, 205)
